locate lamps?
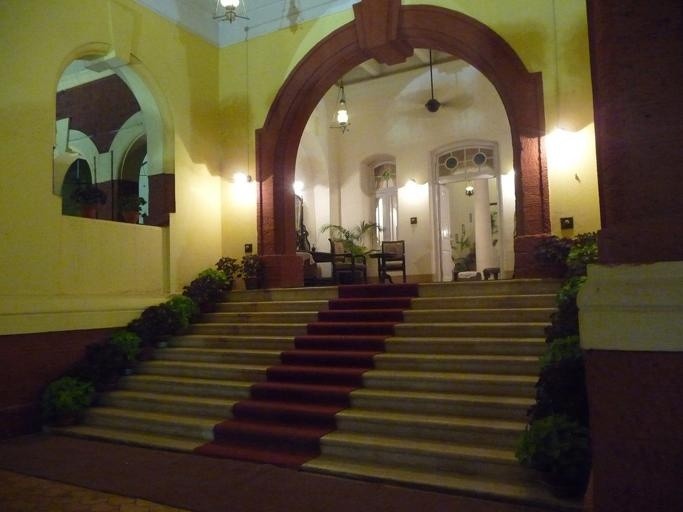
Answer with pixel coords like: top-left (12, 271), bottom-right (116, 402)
top-left (329, 77), bottom-right (351, 134)
top-left (465, 181), bottom-right (475, 196)
top-left (425, 49), bottom-right (440, 111)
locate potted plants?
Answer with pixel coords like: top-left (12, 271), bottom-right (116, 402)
top-left (514, 232), bottom-right (599, 499)
top-left (72, 186), bottom-right (106, 218)
top-left (39, 255), bottom-right (264, 425)
top-left (114, 192), bottom-right (147, 223)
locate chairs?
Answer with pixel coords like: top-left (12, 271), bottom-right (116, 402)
top-left (328, 238), bottom-right (406, 283)
